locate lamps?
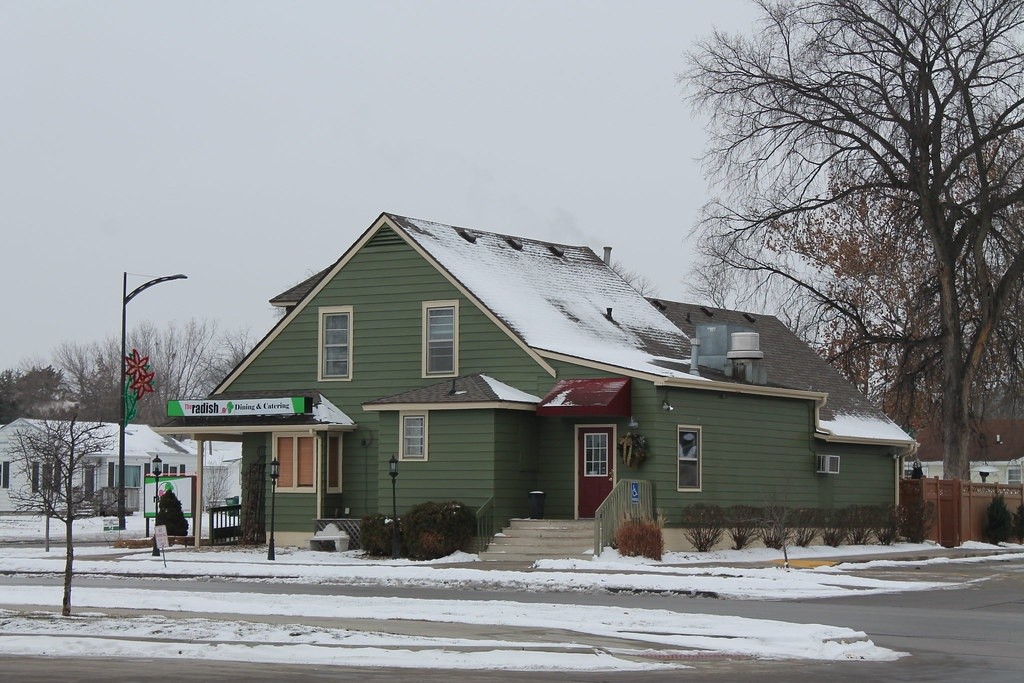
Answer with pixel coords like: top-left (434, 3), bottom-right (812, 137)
top-left (663, 401), bottom-right (673, 411)
top-left (629, 416), bottom-right (638, 429)
top-left (887, 452), bottom-right (897, 459)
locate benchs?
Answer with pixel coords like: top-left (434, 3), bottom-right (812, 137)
top-left (306, 523), bottom-right (349, 551)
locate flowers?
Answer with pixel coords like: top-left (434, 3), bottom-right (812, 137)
top-left (617, 432), bottom-right (649, 466)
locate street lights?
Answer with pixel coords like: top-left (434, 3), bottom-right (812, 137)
top-left (118, 269), bottom-right (188, 530)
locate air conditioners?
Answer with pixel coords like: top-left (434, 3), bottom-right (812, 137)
top-left (815, 454), bottom-right (840, 474)
